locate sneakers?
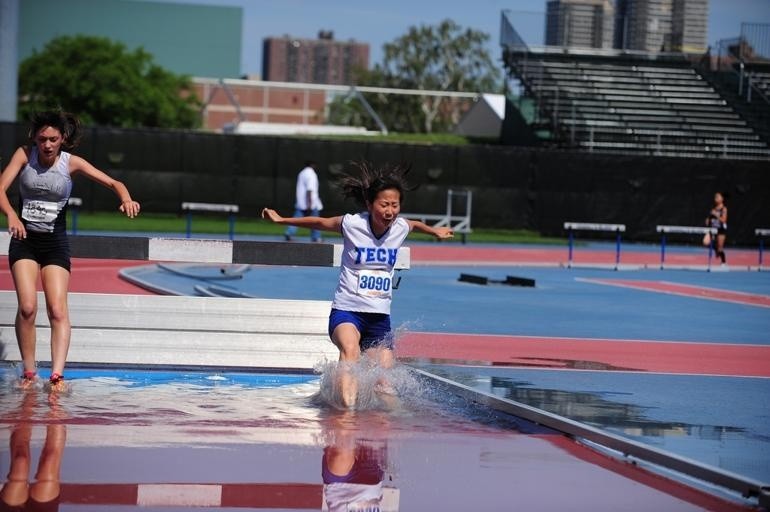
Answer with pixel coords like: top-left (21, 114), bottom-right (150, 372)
top-left (15, 370), bottom-right (41, 393)
top-left (715, 251), bottom-right (727, 267)
top-left (48, 375), bottom-right (68, 396)
top-left (310, 237), bottom-right (323, 243)
top-left (283, 233), bottom-right (292, 241)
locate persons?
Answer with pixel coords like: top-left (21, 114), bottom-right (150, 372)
top-left (0, 111), bottom-right (141, 392)
top-left (319, 408), bottom-right (399, 512)
top-left (701, 192), bottom-right (729, 267)
top-left (283, 159), bottom-right (325, 244)
top-left (1, 380), bottom-right (75, 511)
top-left (262, 153), bottom-right (454, 408)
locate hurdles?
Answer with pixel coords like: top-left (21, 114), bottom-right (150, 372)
top-left (755, 228), bottom-right (770, 271)
top-left (656, 225), bottom-right (717, 273)
top-left (564, 222), bottom-right (625, 270)
top-left (0, 229), bottom-right (411, 370)
top-left (68, 198), bottom-right (82, 235)
top-left (182, 202), bottom-right (239, 240)
